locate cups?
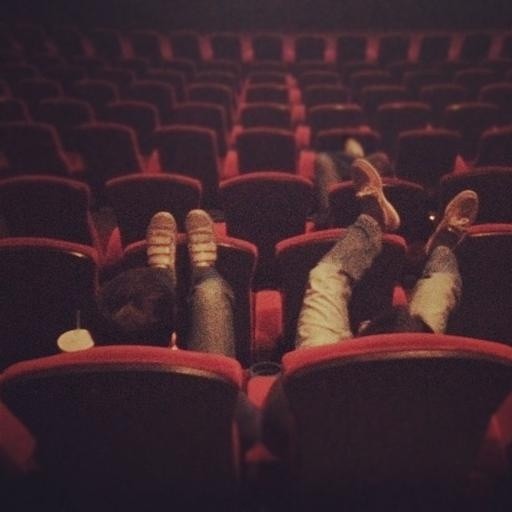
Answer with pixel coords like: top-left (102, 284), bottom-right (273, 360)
top-left (55, 327), bottom-right (96, 354)
top-left (249, 360), bottom-right (281, 378)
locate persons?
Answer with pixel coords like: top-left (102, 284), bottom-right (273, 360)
top-left (311, 138), bottom-right (395, 226)
top-left (288, 158), bottom-right (480, 352)
top-left (93, 208), bottom-right (236, 362)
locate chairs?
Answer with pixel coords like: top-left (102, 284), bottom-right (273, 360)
top-left (1, 27), bottom-right (512, 511)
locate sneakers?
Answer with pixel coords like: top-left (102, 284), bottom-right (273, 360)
top-left (351, 156), bottom-right (401, 233)
top-left (424, 190), bottom-right (479, 255)
top-left (145, 211), bottom-right (177, 288)
top-left (185, 210), bottom-right (217, 269)
